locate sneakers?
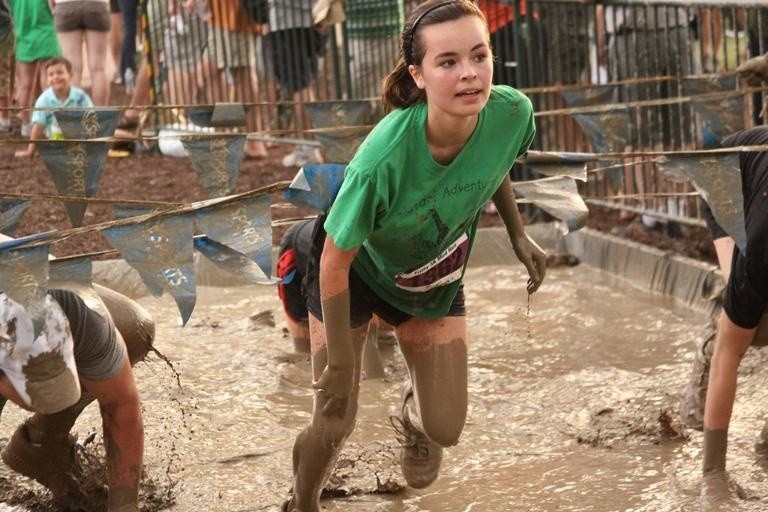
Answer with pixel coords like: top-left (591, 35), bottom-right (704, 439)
top-left (1, 421), bottom-right (84, 494)
top-left (399, 387), bottom-right (443, 489)
top-left (680, 329), bottom-right (716, 431)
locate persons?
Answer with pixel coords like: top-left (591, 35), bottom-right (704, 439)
top-left (284, 0), bottom-right (550, 511)
top-left (135, 1), bottom-right (325, 168)
top-left (0, 230), bottom-right (157, 512)
top-left (247, 213), bottom-right (399, 378)
top-left (0, 0), bottom-right (137, 158)
top-left (693, 121), bottom-right (768, 503)
top-left (332, 0), bottom-right (768, 156)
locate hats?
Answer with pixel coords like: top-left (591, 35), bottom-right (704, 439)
top-left (0, 291), bottom-right (82, 415)
